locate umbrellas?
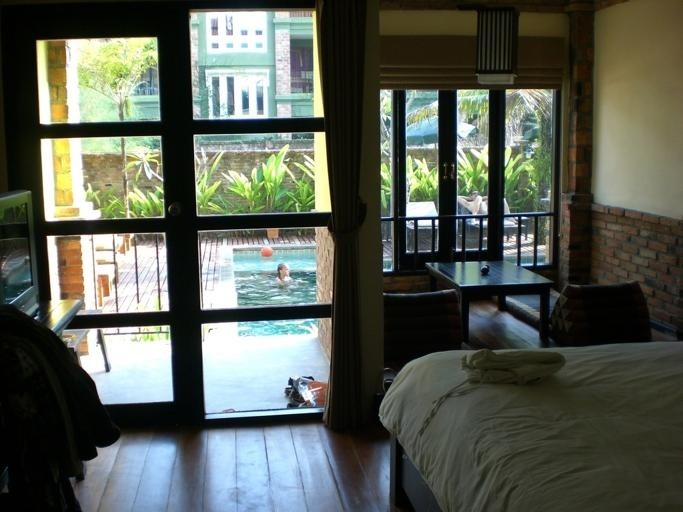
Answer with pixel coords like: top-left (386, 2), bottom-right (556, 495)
top-left (405, 114), bottom-right (478, 146)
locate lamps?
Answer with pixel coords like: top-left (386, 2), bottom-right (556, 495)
top-left (474, 6), bottom-right (518, 85)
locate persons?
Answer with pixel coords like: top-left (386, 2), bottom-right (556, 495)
top-left (467, 191), bottom-right (487, 223)
top-left (272, 261), bottom-right (294, 289)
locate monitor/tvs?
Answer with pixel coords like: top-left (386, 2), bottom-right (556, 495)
top-left (0, 188), bottom-right (40, 320)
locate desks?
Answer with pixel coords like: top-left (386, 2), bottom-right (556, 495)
top-left (99, 264), bottom-right (115, 297)
top-left (41, 300), bottom-right (83, 340)
top-left (425, 260), bottom-right (555, 341)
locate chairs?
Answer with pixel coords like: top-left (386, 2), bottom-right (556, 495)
top-left (555, 281), bottom-right (650, 346)
top-left (459, 199), bottom-right (525, 242)
top-left (406, 201), bottom-right (438, 250)
top-left (381, 289), bottom-right (464, 394)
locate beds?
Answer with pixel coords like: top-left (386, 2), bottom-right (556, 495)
top-left (377, 341), bottom-right (683, 512)
